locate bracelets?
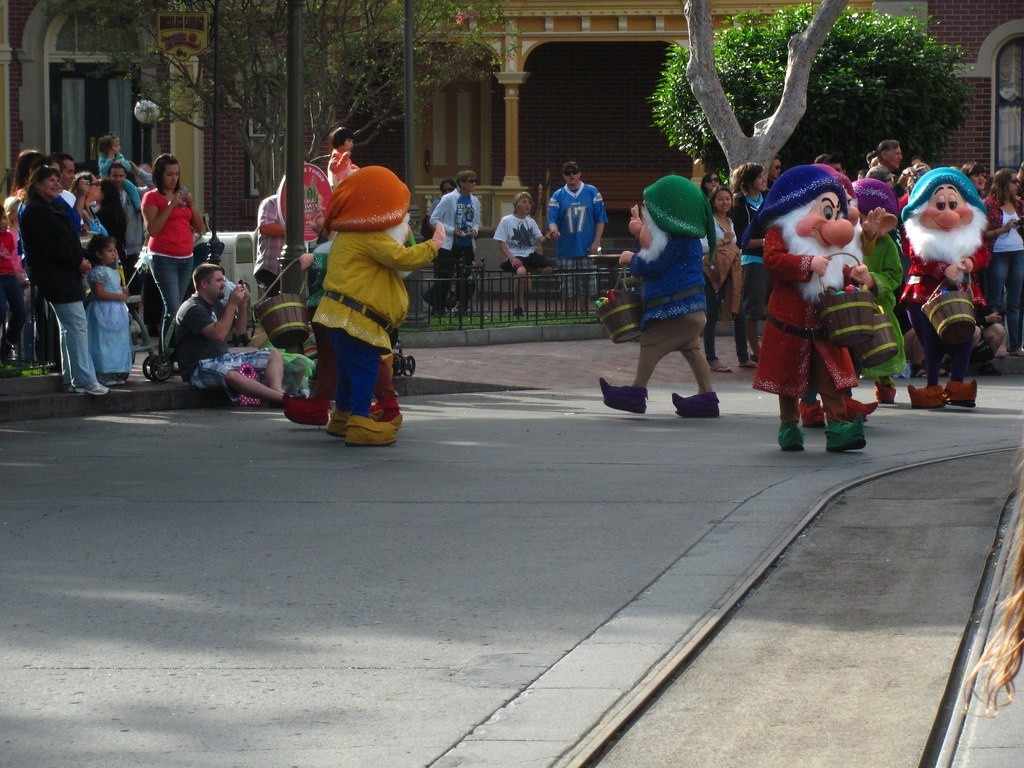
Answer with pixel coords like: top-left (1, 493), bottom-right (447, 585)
top-left (721, 240), bottom-right (726, 245)
top-left (762, 238), bottom-right (765, 247)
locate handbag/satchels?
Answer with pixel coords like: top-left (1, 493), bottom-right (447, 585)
top-left (420, 215), bottom-right (433, 238)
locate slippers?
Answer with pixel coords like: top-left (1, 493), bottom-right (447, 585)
top-left (711, 365), bottom-right (733, 372)
top-left (739, 363), bottom-right (758, 368)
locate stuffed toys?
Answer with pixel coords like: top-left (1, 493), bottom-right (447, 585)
top-left (742, 164), bottom-right (992, 452)
top-left (284, 166), bottom-right (445, 447)
top-left (598, 175), bottom-right (720, 417)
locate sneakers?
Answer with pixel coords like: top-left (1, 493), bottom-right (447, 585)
top-left (63, 381), bottom-right (110, 396)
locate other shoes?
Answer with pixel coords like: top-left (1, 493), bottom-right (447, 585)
top-left (994, 350), bottom-right (1009, 357)
top-left (750, 352), bottom-right (758, 361)
top-left (970, 355), bottom-right (1002, 375)
top-left (911, 364), bottom-right (927, 378)
top-left (939, 366), bottom-right (951, 377)
top-left (1009, 347), bottom-right (1024, 356)
top-left (513, 307), bottom-right (523, 316)
top-left (98, 373), bottom-right (125, 385)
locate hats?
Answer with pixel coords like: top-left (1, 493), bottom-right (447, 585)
top-left (561, 161), bottom-right (580, 171)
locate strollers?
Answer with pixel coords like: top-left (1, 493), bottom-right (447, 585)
top-left (79, 200), bottom-right (173, 383)
top-left (182, 242), bottom-right (252, 347)
top-left (392, 336), bottom-right (416, 376)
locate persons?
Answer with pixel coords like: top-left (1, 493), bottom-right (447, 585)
top-left (493, 192), bottom-right (555, 317)
top-left (426, 169), bottom-right (481, 319)
top-left (174, 263), bottom-right (306, 409)
top-left (700, 140), bottom-right (1024, 378)
top-left (328, 128), bottom-right (360, 193)
top-left (545, 162), bottom-right (608, 316)
top-left (254, 194), bottom-right (287, 298)
top-left (0, 135), bottom-right (205, 395)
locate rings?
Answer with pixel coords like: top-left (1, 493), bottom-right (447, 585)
top-left (1011, 222), bottom-right (1013, 225)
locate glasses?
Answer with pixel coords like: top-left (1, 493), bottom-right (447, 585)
top-left (706, 177), bottom-right (719, 182)
top-left (91, 181), bottom-right (103, 186)
top-left (465, 179), bottom-right (478, 183)
top-left (563, 170), bottom-right (579, 175)
top-left (1010, 178), bottom-right (1019, 184)
top-left (772, 165), bottom-right (781, 169)
top-left (441, 188), bottom-right (454, 192)
top-left (37, 165), bottom-right (48, 180)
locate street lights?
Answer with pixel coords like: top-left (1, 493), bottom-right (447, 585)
top-left (134, 99), bottom-right (160, 166)
